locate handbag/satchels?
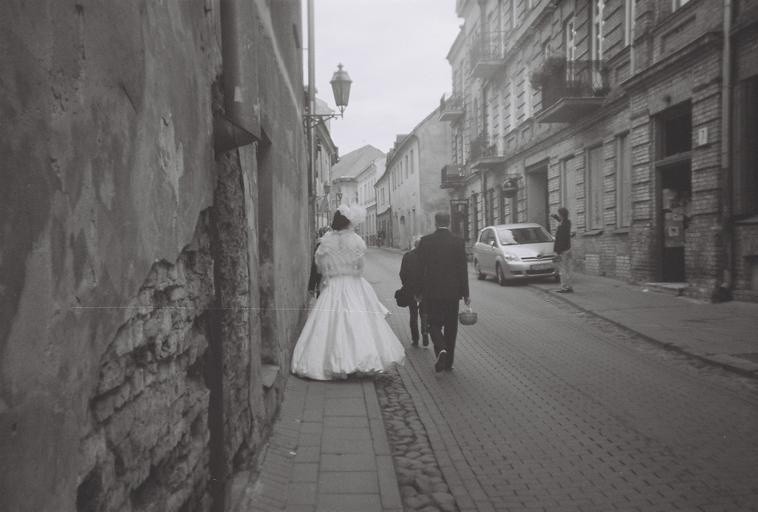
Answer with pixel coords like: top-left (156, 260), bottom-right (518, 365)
top-left (459, 310), bottom-right (478, 326)
top-left (394, 288), bottom-right (411, 307)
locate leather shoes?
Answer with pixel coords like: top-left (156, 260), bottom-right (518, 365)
top-left (434, 350), bottom-right (450, 373)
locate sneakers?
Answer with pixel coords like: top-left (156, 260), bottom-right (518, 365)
top-left (555, 288), bottom-right (575, 292)
top-left (421, 332), bottom-right (429, 347)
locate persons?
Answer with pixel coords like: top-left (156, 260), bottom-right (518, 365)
top-left (290, 201), bottom-right (409, 383)
top-left (306, 225), bottom-right (331, 301)
top-left (415, 209), bottom-right (472, 374)
top-left (550, 207), bottom-right (575, 293)
top-left (400, 233), bottom-right (430, 350)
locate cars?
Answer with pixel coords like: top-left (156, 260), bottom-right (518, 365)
top-left (471, 222), bottom-right (562, 285)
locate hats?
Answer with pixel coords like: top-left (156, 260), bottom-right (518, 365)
top-left (337, 202), bottom-right (367, 226)
